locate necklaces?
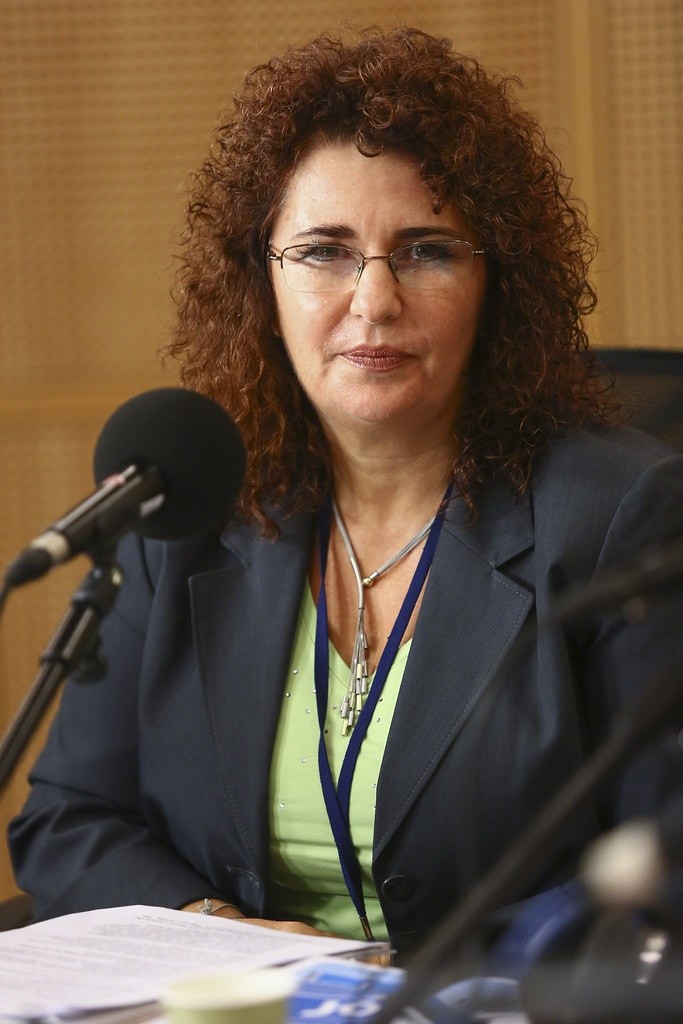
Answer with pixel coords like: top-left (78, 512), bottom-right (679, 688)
top-left (310, 444), bottom-right (460, 736)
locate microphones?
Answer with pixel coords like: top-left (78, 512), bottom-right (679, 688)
top-left (342, 604), bottom-right (680, 1022)
top-left (2, 388), bottom-right (248, 592)
top-left (523, 541), bottom-right (683, 651)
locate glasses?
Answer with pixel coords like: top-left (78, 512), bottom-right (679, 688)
top-left (267, 240), bottom-right (487, 293)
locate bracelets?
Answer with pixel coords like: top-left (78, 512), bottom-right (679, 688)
top-left (194, 897), bottom-right (237, 920)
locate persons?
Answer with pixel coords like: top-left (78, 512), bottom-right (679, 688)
top-left (5, 29), bottom-right (681, 973)
